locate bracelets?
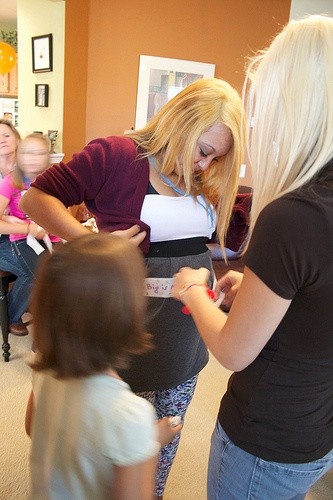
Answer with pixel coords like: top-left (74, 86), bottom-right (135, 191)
top-left (178, 283), bottom-right (207, 301)
top-left (25, 222), bottom-right (30, 235)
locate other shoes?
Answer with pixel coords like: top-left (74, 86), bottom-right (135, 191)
top-left (7, 318), bottom-right (29, 336)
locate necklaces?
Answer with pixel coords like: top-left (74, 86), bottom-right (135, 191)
top-left (150, 157), bottom-right (215, 229)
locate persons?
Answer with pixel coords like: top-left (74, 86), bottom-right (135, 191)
top-left (16, 77), bottom-right (249, 498)
top-left (0, 131), bottom-right (64, 282)
top-left (0, 119), bottom-right (47, 337)
top-left (170, 14), bottom-right (333, 500)
top-left (22, 232), bottom-right (184, 499)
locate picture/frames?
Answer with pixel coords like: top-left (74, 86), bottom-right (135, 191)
top-left (31, 33), bottom-right (53, 74)
top-left (35, 83), bottom-right (49, 107)
top-left (135, 54), bottom-right (216, 131)
